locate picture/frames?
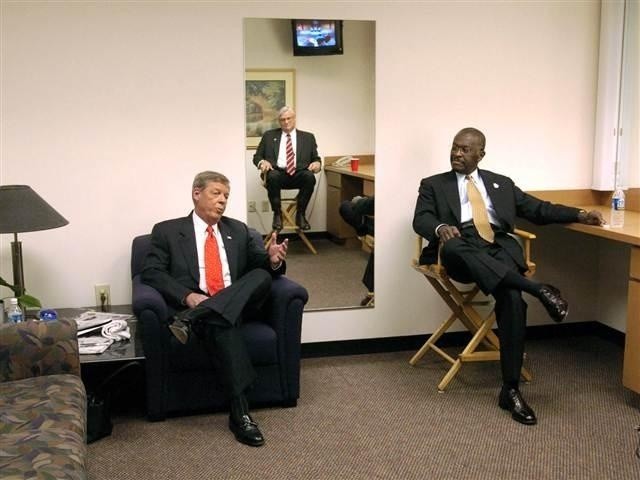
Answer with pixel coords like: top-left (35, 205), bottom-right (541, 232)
top-left (244, 68), bottom-right (297, 149)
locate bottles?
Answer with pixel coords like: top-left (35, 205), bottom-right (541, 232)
top-left (610, 187), bottom-right (625, 228)
top-left (7, 298), bottom-right (23, 325)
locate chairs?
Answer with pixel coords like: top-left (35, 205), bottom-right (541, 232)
top-left (258, 168), bottom-right (318, 255)
top-left (408, 224), bottom-right (537, 393)
top-left (357, 235), bottom-right (373, 307)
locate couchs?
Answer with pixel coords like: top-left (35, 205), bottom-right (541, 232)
top-left (0, 322), bottom-right (87, 480)
top-left (131, 233), bottom-right (311, 421)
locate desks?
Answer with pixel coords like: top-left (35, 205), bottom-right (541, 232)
top-left (506, 188), bottom-right (640, 247)
top-left (325, 155), bottom-right (375, 182)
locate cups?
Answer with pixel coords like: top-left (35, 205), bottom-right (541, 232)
top-left (351, 159), bottom-right (359, 172)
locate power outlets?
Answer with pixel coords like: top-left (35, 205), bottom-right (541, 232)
top-left (95, 285), bottom-right (110, 305)
top-left (248, 201), bottom-right (256, 212)
top-left (261, 201), bottom-right (269, 212)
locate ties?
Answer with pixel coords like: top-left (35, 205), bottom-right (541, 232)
top-left (286, 134), bottom-right (296, 176)
top-left (467, 174), bottom-right (495, 244)
top-left (204, 226), bottom-right (225, 296)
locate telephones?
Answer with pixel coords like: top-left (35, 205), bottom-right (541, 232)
top-left (332, 156), bottom-right (352, 167)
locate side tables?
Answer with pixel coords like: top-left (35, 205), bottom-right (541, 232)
top-left (29, 303), bottom-right (147, 444)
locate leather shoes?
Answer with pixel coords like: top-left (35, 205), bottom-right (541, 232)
top-left (360, 295), bottom-right (371, 305)
top-left (296, 214), bottom-right (311, 230)
top-left (228, 413), bottom-right (263, 446)
top-left (499, 386), bottom-right (537, 425)
top-left (168, 311), bottom-right (191, 345)
top-left (537, 284), bottom-right (569, 322)
top-left (272, 211), bottom-right (282, 229)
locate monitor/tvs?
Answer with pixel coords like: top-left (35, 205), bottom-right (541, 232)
top-left (292, 19), bottom-right (344, 56)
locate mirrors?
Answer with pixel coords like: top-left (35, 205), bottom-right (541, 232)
top-left (243, 16), bottom-right (377, 310)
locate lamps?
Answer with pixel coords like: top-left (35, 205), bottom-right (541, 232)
top-left (0, 186), bottom-right (69, 321)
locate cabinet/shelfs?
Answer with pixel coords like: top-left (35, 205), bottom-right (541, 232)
top-left (325, 171), bottom-right (363, 245)
top-left (619, 244), bottom-right (640, 394)
top-left (362, 180), bottom-right (374, 251)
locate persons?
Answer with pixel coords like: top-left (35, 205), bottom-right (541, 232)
top-left (140, 170), bottom-right (289, 445)
top-left (339, 195), bottom-right (375, 306)
top-left (252, 107), bottom-right (321, 231)
top-left (413, 127), bottom-right (606, 425)
